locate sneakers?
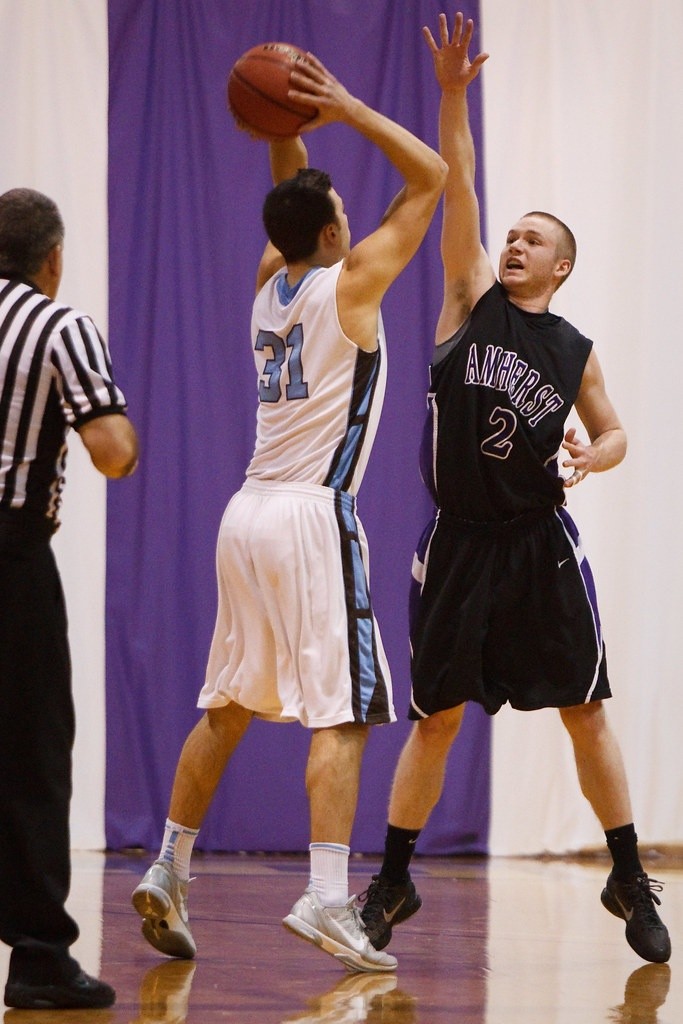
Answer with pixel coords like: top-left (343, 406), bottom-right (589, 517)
top-left (601, 865), bottom-right (671, 963)
top-left (358, 870), bottom-right (422, 950)
top-left (284, 886), bottom-right (398, 971)
top-left (131, 858), bottom-right (197, 957)
top-left (4, 945), bottom-right (115, 1010)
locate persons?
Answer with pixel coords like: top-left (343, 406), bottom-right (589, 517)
top-left (133, 50), bottom-right (448, 971)
top-left (0, 188), bottom-right (138, 1008)
top-left (358, 11), bottom-right (672, 962)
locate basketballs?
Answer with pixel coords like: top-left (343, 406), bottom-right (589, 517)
top-left (229, 44), bottom-right (322, 142)
